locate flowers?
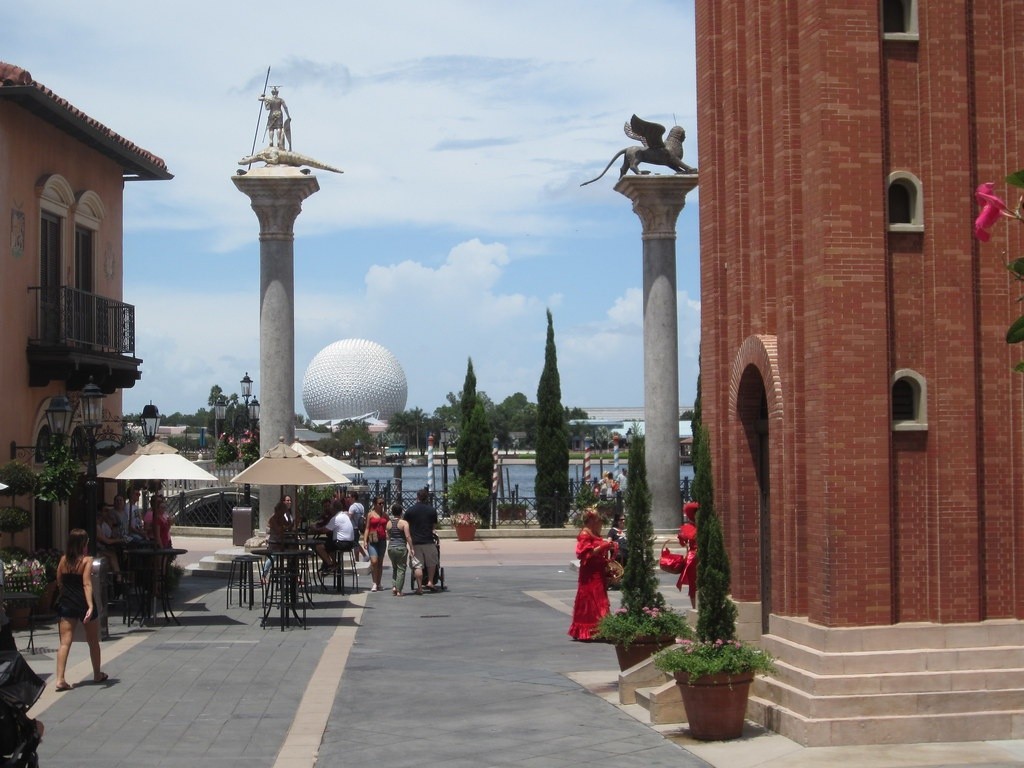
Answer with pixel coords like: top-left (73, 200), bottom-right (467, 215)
top-left (973, 171), bottom-right (1024, 374)
top-left (451, 512), bottom-right (483, 529)
top-left (588, 603), bottom-right (696, 653)
top-left (653, 634), bottom-right (783, 687)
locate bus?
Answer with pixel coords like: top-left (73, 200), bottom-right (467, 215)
top-left (389, 444), bottom-right (406, 453)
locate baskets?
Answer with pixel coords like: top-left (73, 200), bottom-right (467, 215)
top-left (659, 539), bottom-right (687, 574)
top-left (606, 561), bottom-right (625, 586)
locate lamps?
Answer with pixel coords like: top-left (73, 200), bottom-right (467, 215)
top-left (236, 169), bottom-right (246, 175)
top-left (300, 168), bottom-right (310, 175)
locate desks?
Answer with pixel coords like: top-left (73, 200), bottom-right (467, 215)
top-left (124, 548), bottom-right (187, 625)
top-left (260, 551), bottom-right (313, 629)
top-left (282, 539), bottom-right (325, 590)
top-left (0, 592), bottom-right (39, 599)
top-left (284, 530), bottom-right (331, 586)
top-left (113, 540), bottom-right (155, 599)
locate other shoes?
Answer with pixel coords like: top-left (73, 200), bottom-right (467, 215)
top-left (322, 563), bottom-right (340, 575)
top-left (416, 589), bottom-right (423, 595)
top-left (259, 577), bottom-right (269, 584)
top-left (426, 584), bottom-right (437, 591)
top-left (392, 587), bottom-right (405, 596)
top-left (371, 583), bottom-right (383, 592)
top-left (297, 580), bottom-right (305, 584)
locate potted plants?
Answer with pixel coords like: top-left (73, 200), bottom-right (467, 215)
top-left (596, 500), bottom-right (618, 518)
top-left (496, 503), bottom-right (528, 520)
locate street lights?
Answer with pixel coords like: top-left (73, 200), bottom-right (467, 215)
top-left (46, 375), bottom-right (107, 557)
top-left (354, 438), bottom-right (365, 484)
top-left (626, 429), bottom-right (634, 466)
top-left (440, 425), bottom-right (450, 517)
top-left (214, 373), bottom-right (260, 507)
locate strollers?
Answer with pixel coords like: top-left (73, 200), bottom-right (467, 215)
top-left (410, 534), bottom-right (446, 591)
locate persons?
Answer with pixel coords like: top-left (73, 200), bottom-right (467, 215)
top-left (371, 452), bottom-right (402, 463)
top-left (95, 485), bottom-right (175, 600)
top-left (568, 467), bottom-right (698, 641)
top-left (263, 87), bottom-right (291, 147)
top-left (0, 606), bottom-right (46, 768)
top-left (56, 528), bottom-right (108, 689)
top-left (260, 489), bottom-right (440, 596)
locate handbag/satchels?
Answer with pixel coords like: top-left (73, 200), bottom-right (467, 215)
top-left (368, 531), bottom-right (379, 543)
top-left (53, 593), bottom-right (64, 609)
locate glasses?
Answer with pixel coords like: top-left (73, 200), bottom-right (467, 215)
top-left (619, 518), bottom-right (625, 521)
top-left (376, 502), bottom-right (384, 505)
top-left (134, 492), bottom-right (141, 496)
top-left (285, 499), bottom-right (292, 502)
top-left (103, 508), bottom-right (109, 512)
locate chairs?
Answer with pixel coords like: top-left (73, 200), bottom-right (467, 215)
top-left (26, 519), bottom-right (365, 654)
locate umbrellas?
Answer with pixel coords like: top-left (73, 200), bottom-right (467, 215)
top-left (231, 435), bottom-right (364, 533)
top-left (84, 437), bottom-right (218, 535)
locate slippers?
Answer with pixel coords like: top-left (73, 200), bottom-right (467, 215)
top-left (55, 682), bottom-right (73, 692)
top-left (93, 672), bottom-right (108, 683)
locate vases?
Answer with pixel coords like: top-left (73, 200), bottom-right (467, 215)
top-left (614, 635), bottom-right (677, 671)
top-left (672, 671), bottom-right (754, 741)
top-left (455, 524), bottom-right (477, 541)
